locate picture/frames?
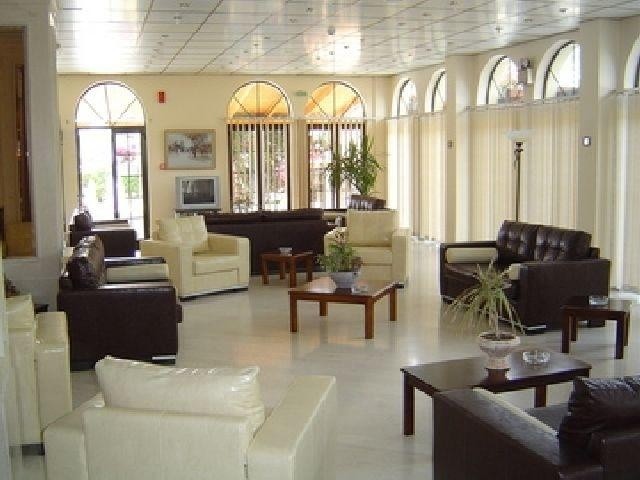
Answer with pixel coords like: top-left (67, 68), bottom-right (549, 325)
top-left (164, 128), bottom-right (216, 170)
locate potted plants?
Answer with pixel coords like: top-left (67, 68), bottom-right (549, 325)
top-left (439, 256), bottom-right (527, 370)
top-left (316, 230), bottom-right (364, 288)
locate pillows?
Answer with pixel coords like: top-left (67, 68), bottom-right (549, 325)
top-left (557, 375), bottom-right (640, 438)
top-left (157, 216), bottom-right (210, 253)
top-left (348, 208), bottom-right (400, 247)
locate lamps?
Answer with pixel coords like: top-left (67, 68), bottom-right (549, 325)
top-left (506, 129), bottom-right (534, 221)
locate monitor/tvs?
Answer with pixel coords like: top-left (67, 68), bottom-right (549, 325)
top-left (174, 175), bottom-right (222, 211)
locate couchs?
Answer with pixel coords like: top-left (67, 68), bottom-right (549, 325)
top-left (139, 217), bottom-right (251, 302)
top-left (325, 209), bottom-right (412, 289)
top-left (56, 234), bottom-right (183, 373)
top-left (40, 356), bottom-right (336, 480)
top-left (5, 294), bottom-right (73, 454)
top-left (433, 378), bottom-right (640, 480)
top-left (68, 206), bottom-right (140, 256)
top-left (199, 208), bottom-right (336, 274)
top-left (323, 194), bottom-right (389, 227)
top-left (440, 221), bottom-right (611, 335)
